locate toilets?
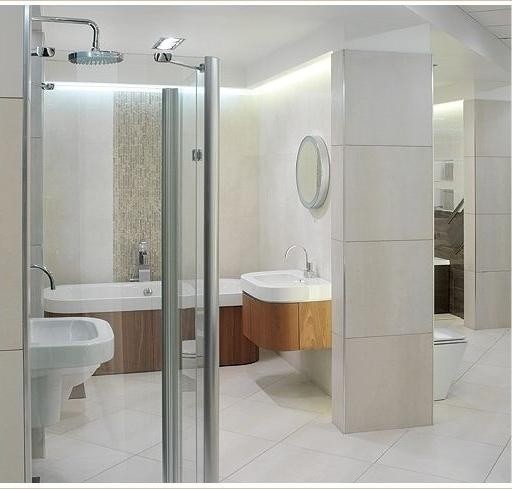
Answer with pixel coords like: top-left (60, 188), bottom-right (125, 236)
top-left (432, 328), bottom-right (467, 401)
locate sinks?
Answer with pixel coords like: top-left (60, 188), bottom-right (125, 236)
top-left (242, 270), bottom-right (332, 303)
top-left (30, 317), bottom-right (115, 379)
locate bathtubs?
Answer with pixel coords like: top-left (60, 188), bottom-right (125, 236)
top-left (43, 278), bottom-right (243, 314)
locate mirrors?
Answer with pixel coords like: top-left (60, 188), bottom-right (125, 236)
top-left (295, 135), bottom-right (330, 210)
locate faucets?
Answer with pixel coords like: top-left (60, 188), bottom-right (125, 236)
top-left (139, 242), bottom-right (147, 263)
top-left (284, 245), bottom-right (309, 271)
top-left (30, 264), bottom-right (56, 290)
top-left (144, 287), bottom-right (153, 296)
